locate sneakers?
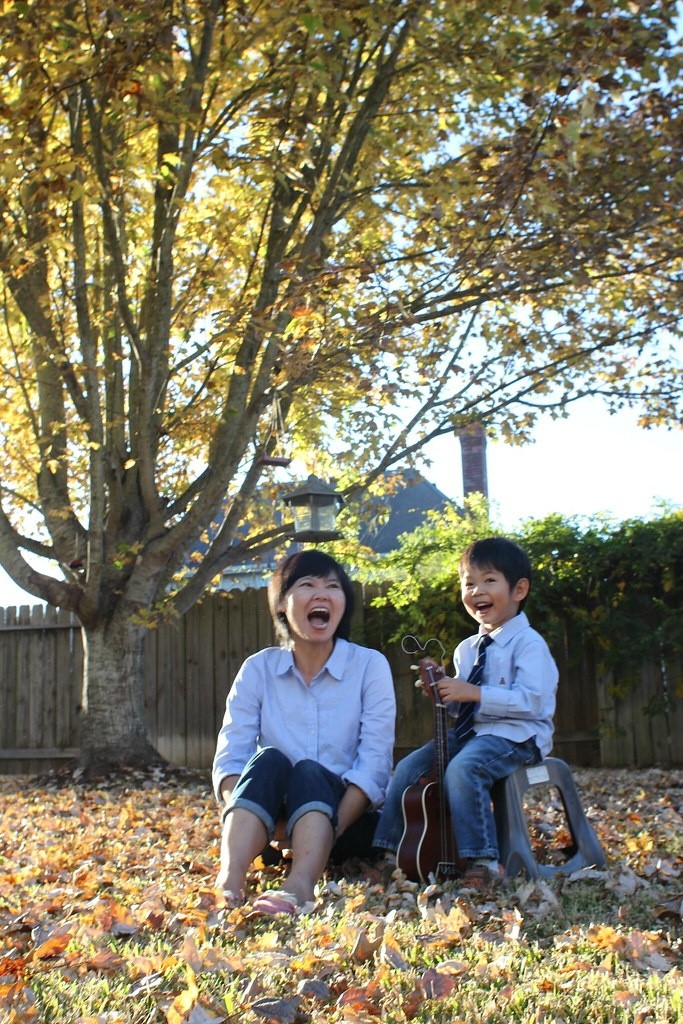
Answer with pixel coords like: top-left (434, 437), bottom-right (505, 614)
top-left (362, 862), bottom-right (397, 884)
top-left (459, 865), bottom-right (511, 897)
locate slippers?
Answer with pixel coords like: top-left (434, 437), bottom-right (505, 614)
top-left (251, 890), bottom-right (297, 914)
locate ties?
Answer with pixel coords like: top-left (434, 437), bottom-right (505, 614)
top-left (455, 634), bottom-right (495, 743)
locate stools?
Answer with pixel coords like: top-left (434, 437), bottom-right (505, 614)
top-left (489, 758), bottom-right (606, 882)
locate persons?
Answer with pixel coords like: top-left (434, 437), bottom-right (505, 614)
top-left (169, 550), bottom-right (396, 941)
top-left (344, 537), bottom-right (559, 893)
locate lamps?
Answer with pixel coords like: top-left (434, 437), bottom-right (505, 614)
top-left (283, 473), bottom-right (347, 541)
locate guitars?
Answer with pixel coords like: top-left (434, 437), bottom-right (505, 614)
top-left (396, 656), bottom-right (469, 886)
